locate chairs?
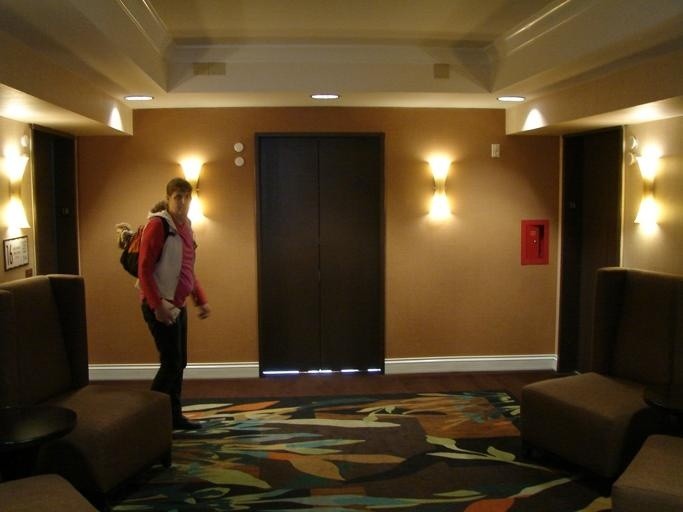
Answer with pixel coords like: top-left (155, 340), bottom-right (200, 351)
top-left (0, 272), bottom-right (174, 494)
top-left (522, 264), bottom-right (683, 495)
top-left (611, 434), bottom-right (683, 512)
top-left (0, 473), bottom-right (99, 512)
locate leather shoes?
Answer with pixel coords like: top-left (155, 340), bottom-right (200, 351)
top-left (175, 420), bottom-right (202, 429)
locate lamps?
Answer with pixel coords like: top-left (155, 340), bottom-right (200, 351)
top-left (179, 163), bottom-right (205, 215)
top-left (428, 159), bottom-right (452, 214)
top-left (634, 156), bottom-right (663, 224)
top-left (2, 156), bottom-right (31, 229)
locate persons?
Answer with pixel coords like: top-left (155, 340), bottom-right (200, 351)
top-left (135, 178), bottom-right (213, 437)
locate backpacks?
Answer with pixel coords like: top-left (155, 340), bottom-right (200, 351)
top-left (120, 216), bottom-right (170, 278)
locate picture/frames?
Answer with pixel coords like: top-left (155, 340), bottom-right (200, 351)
top-left (3, 234), bottom-right (29, 271)
